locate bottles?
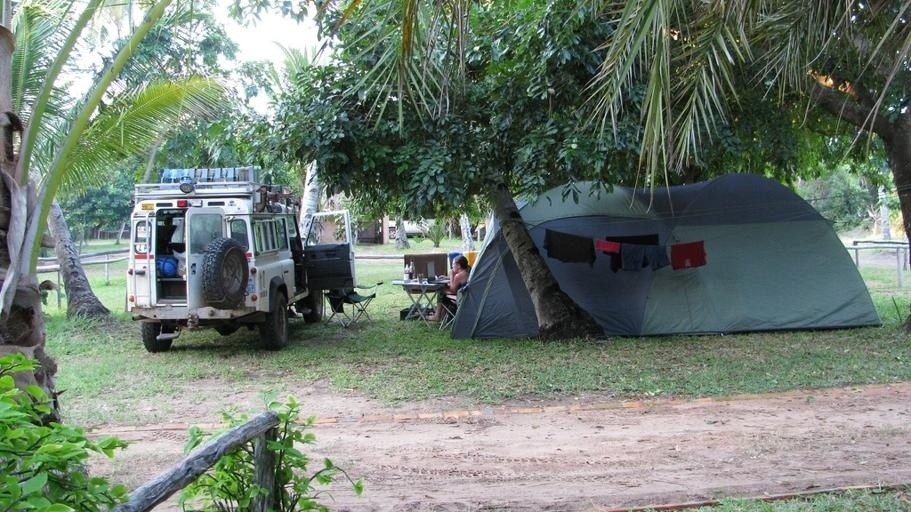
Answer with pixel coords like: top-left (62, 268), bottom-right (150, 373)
top-left (409, 259), bottom-right (415, 279)
top-left (403, 264), bottom-right (410, 283)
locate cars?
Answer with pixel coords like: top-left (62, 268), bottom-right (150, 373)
top-left (389, 216), bottom-right (435, 239)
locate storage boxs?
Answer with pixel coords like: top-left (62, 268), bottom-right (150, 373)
top-left (155, 250), bottom-right (186, 279)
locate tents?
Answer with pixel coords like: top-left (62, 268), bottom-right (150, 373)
top-left (450, 173), bottom-right (883, 339)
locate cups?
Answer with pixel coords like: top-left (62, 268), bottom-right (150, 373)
top-left (422, 279), bottom-right (427, 284)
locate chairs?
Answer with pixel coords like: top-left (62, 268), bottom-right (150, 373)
top-left (325, 281), bottom-right (383, 331)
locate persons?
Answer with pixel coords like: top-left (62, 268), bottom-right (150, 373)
top-left (426, 255), bottom-right (470, 321)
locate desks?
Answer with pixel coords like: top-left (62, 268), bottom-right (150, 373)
top-left (392, 272), bottom-right (468, 332)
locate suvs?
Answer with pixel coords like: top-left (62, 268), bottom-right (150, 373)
top-left (125, 166), bottom-right (358, 352)
